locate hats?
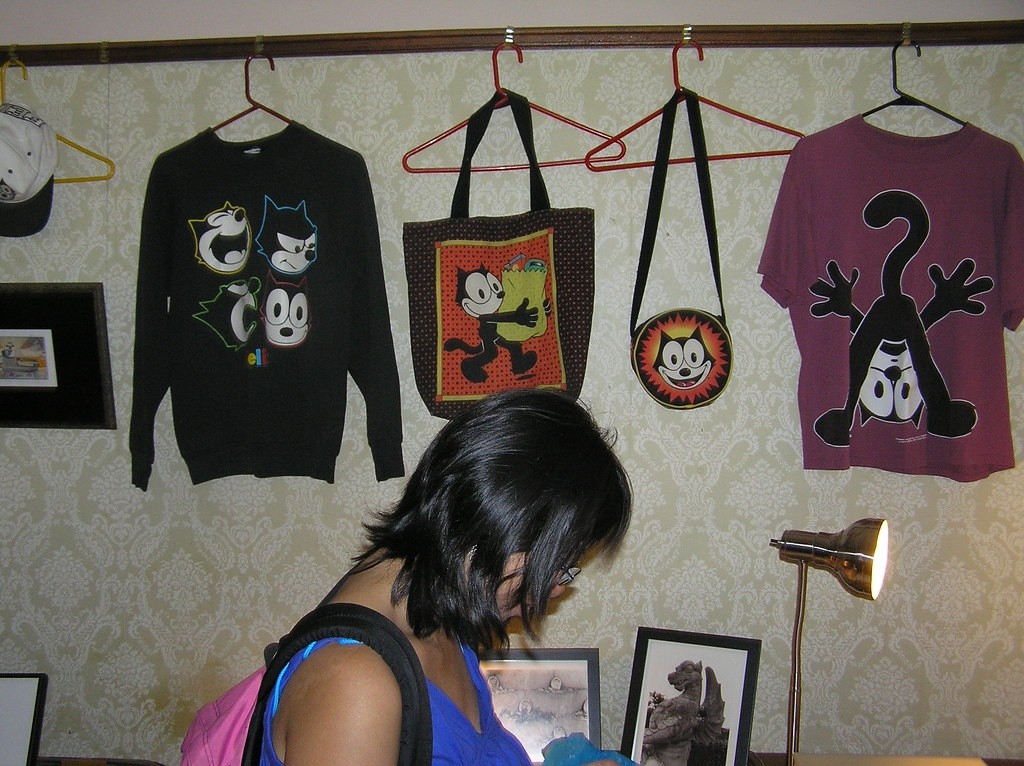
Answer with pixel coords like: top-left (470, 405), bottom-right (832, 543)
top-left (0, 104), bottom-right (59, 236)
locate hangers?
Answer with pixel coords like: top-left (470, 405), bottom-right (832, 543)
top-left (212, 53), bottom-right (292, 132)
top-left (402, 43), bottom-right (627, 173)
top-left (862, 40), bottom-right (967, 128)
top-left (0, 59), bottom-right (115, 185)
top-left (585, 40), bottom-right (804, 173)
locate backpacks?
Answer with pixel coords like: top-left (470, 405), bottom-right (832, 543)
top-left (181, 603), bottom-right (432, 766)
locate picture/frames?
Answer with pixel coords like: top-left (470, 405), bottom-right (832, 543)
top-left (0, 283), bottom-right (118, 430)
top-left (475, 648), bottom-right (601, 766)
top-left (0, 673), bottom-right (50, 766)
top-left (620, 626), bottom-right (762, 766)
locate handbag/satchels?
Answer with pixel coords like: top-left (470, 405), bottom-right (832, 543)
top-left (630, 308), bottom-right (734, 410)
top-left (402, 87), bottom-right (594, 421)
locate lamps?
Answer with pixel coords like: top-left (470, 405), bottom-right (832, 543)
top-left (770, 518), bottom-right (888, 766)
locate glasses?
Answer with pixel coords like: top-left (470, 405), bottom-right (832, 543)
top-left (558, 564), bottom-right (582, 585)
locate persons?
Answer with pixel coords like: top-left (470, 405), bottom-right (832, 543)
top-left (250, 386), bottom-right (636, 766)
